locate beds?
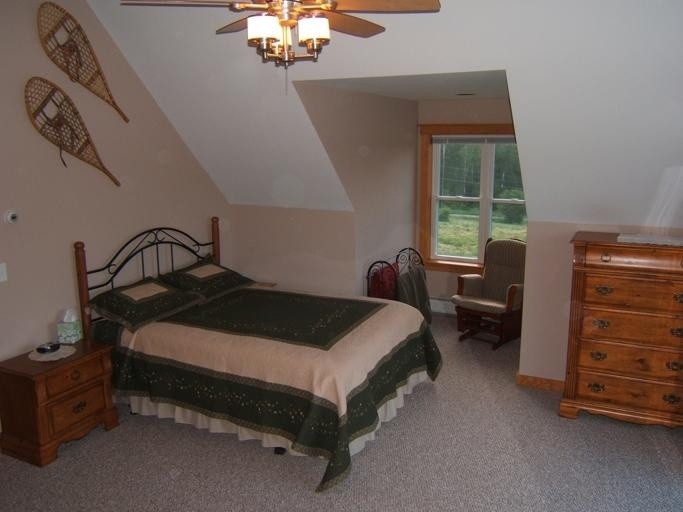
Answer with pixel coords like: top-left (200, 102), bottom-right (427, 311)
top-left (74, 217), bottom-right (442, 492)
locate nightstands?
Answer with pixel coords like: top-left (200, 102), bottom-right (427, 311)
top-left (1, 341), bottom-right (120, 470)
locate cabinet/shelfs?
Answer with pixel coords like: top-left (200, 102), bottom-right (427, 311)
top-left (557, 229), bottom-right (683, 431)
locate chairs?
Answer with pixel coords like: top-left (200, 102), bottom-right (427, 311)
top-left (449, 238), bottom-right (527, 353)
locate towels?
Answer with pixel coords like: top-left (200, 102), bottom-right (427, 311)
top-left (396, 262), bottom-right (433, 326)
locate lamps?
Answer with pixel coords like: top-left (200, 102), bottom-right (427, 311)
top-left (244, 1), bottom-right (331, 68)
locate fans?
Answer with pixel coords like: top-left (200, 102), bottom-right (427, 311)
top-left (119, 0), bottom-right (441, 38)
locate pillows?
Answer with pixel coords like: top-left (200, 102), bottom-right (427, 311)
top-left (160, 258), bottom-right (255, 296)
top-left (87, 276), bottom-right (207, 334)
